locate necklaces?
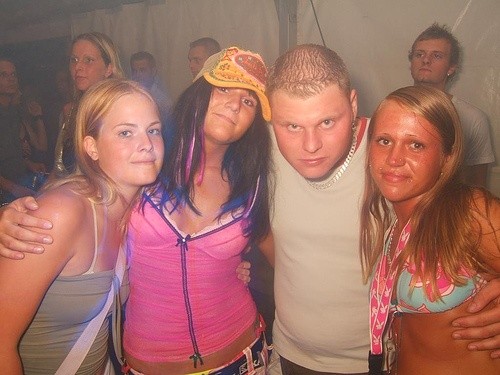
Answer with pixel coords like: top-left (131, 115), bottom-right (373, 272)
top-left (301, 119), bottom-right (358, 190)
top-left (386, 217), bottom-right (398, 268)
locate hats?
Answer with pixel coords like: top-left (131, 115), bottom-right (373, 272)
top-left (192, 47), bottom-right (271, 122)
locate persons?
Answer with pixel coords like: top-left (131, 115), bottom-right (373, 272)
top-left (355, 81), bottom-right (500, 374)
top-left (39, 31), bottom-right (131, 196)
top-left (0, 77), bottom-right (165, 375)
top-left (1, 44), bottom-right (280, 375)
top-left (252, 43), bottom-right (500, 375)
top-left (129, 50), bottom-right (172, 128)
top-left (186, 35), bottom-right (221, 83)
top-left (1, 48), bottom-right (82, 209)
top-left (406, 19), bottom-right (494, 191)
